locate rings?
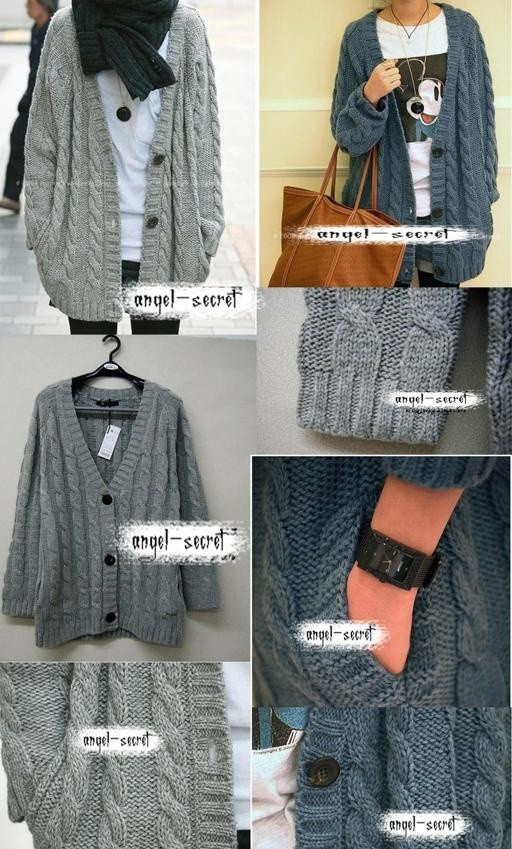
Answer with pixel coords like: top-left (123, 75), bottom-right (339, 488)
top-left (382, 58), bottom-right (393, 70)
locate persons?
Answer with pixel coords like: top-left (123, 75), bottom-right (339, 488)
top-left (24, 0), bottom-right (227, 335)
top-left (252, 456), bottom-right (511, 710)
top-left (1, 0), bottom-right (64, 214)
top-left (328, 0), bottom-right (477, 288)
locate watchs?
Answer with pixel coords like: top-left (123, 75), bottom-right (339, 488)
top-left (352, 524), bottom-right (443, 590)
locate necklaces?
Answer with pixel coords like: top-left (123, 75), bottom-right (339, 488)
top-left (388, 0), bottom-right (429, 44)
top-left (109, 67), bottom-right (137, 121)
top-left (387, 2), bottom-right (429, 108)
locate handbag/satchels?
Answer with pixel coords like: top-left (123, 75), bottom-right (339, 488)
top-left (254, 731), bottom-right (303, 849)
top-left (269, 186), bottom-right (406, 288)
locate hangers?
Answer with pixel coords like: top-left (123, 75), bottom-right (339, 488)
top-left (70, 334), bottom-right (146, 416)
